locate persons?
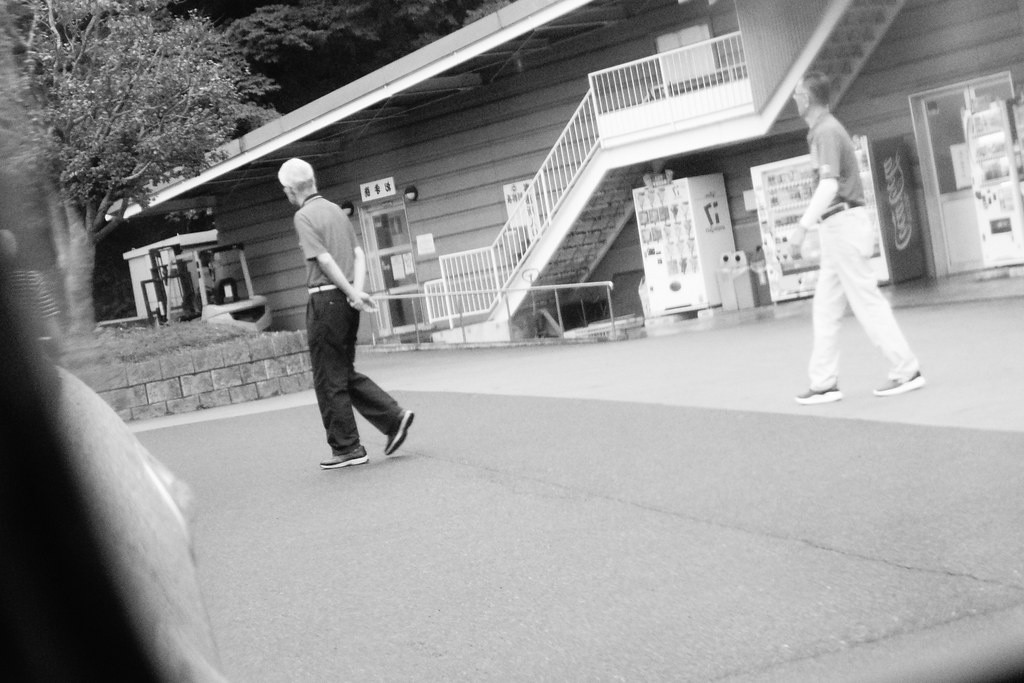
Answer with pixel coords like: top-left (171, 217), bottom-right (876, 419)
top-left (0, 227), bottom-right (66, 360)
top-left (791, 68), bottom-right (924, 406)
top-left (0, 194), bottom-right (229, 683)
top-left (277, 158), bottom-right (415, 470)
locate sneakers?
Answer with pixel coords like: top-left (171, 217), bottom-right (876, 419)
top-left (873, 368), bottom-right (925, 396)
top-left (384, 408), bottom-right (414, 455)
top-left (320, 446), bottom-right (369, 469)
top-left (793, 384), bottom-right (842, 404)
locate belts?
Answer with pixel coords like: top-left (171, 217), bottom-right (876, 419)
top-left (818, 201), bottom-right (862, 223)
top-left (308, 284), bottom-right (354, 295)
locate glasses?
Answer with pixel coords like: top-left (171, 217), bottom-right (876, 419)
top-left (793, 93), bottom-right (804, 99)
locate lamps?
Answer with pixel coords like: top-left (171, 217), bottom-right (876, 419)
top-left (404, 184), bottom-right (418, 202)
top-left (342, 202), bottom-right (355, 217)
